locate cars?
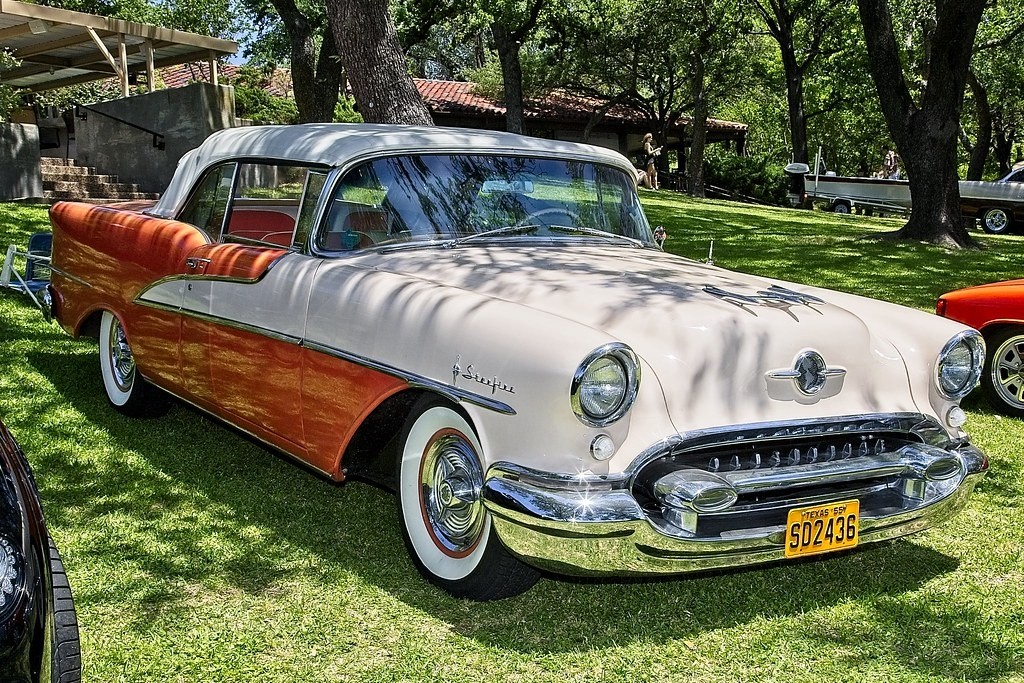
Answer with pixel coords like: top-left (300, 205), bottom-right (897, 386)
top-left (43, 124), bottom-right (989, 600)
top-left (937, 277), bottom-right (1024, 417)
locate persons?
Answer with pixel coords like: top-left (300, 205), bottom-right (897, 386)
top-left (641, 133), bottom-right (663, 191)
top-left (881, 145), bottom-right (903, 179)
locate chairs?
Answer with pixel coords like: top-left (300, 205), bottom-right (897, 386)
top-left (0, 232), bottom-right (55, 309)
top-left (211, 203), bottom-right (536, 252)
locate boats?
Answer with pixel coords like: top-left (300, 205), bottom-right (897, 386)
top-left (804, 173), bottom-right (1024, 224)
